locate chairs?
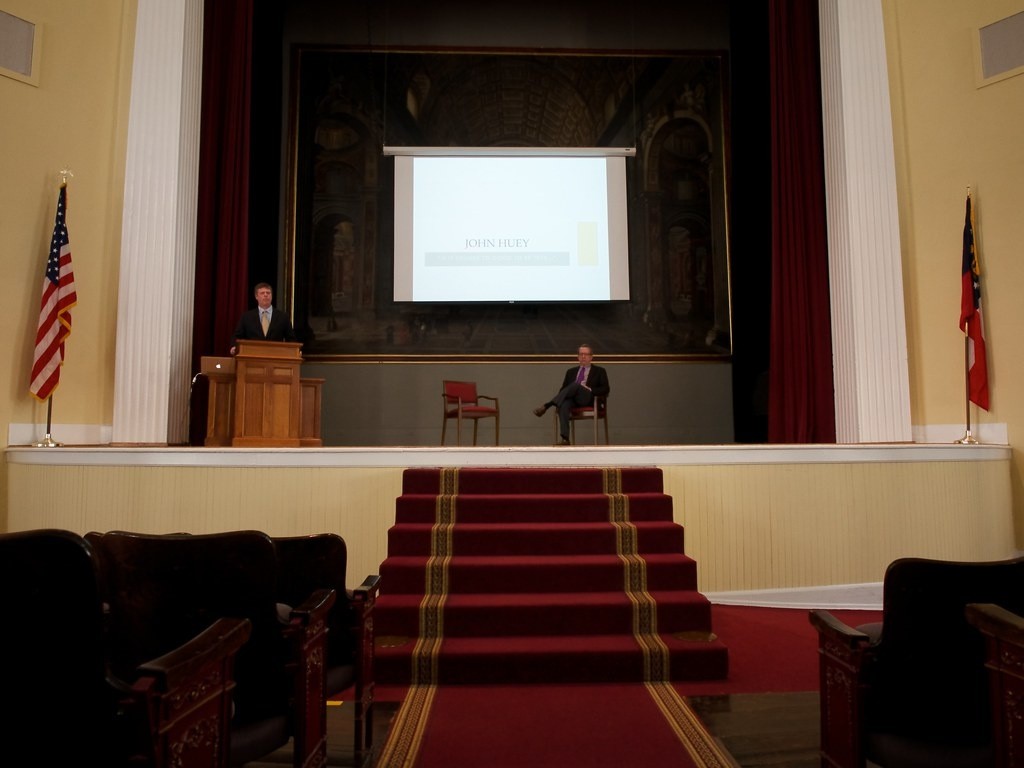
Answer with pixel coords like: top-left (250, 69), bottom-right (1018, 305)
top-left (554, 396), bottom-right (609, 445)
top-left (0, 528), bottom-right (381, 768)
top-left (441, 380), bottom-right (500, 447)
top-left (809, 556), bottom-right (1024, 768)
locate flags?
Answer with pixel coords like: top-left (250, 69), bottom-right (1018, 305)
top-left (959, 195), bottom-right (990, 412)
top-left (28, 181), bottom-right (78, 403)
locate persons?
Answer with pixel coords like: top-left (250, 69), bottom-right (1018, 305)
top-left (225, 282), bottom-right (303, 355)
top-left (533, 343), bottom-right (610, 445)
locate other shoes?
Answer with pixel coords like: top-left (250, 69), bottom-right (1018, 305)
top-left (552, 440), bottom-right (570, 445)
top-left (533, 406), bottom-right (546, 418)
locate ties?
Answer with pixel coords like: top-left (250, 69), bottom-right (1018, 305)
top-left (260, 311), bottom-right (270, 337)
top-left (576, 366), bottom-right (586, 385)
